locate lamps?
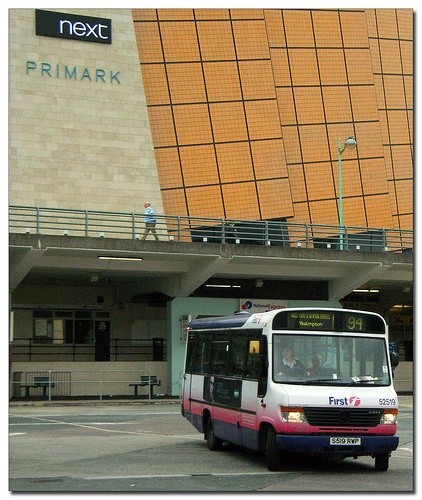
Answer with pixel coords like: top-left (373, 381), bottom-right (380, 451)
top-left (169, 236), bottom-right (174, 241)
top-left (403, 286), bottom-right (410, 293)
top-left (384, 246), bottom-right (389, 251)
top-left (297, 242), bottom-right (302, 247)
top-left (265, 240), bottom-right (270, 246)
top-left (255, 278), bottom-right (263, 287)
top-left (236, 239), bottom-right (240, 244)
top-left (135, 234), bottom-right (140, 240)
top-left (26, 228), bottom-right (31, 233)
top-left (99, 232), bottom-right (104, 237)
top-left (90, 273), bottom-right (100, 281)
top-left (64, 230), bottom-right (68, 235)
top-left (327, 243), bottom-right (331, 249)
top-left (203, 238), bottom-right (208, 243)
top-left (356, 245), bottom-right (361, 251)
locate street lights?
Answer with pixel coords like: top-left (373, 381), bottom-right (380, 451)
top-left (337, 136), bottom-right (359, 251)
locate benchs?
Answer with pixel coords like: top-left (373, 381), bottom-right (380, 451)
top-left (20, 376), bottom-right (55, 398)
top-left (128, 375), bottom-right (162, 398)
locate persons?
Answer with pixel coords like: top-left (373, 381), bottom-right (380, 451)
top-left (139, 201), bottom-right (159, 240)
top-left (272, 342), bottom-right (311, 380)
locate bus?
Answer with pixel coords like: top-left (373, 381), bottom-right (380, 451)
top-left (178, 305), bottom-right (399, 472)
